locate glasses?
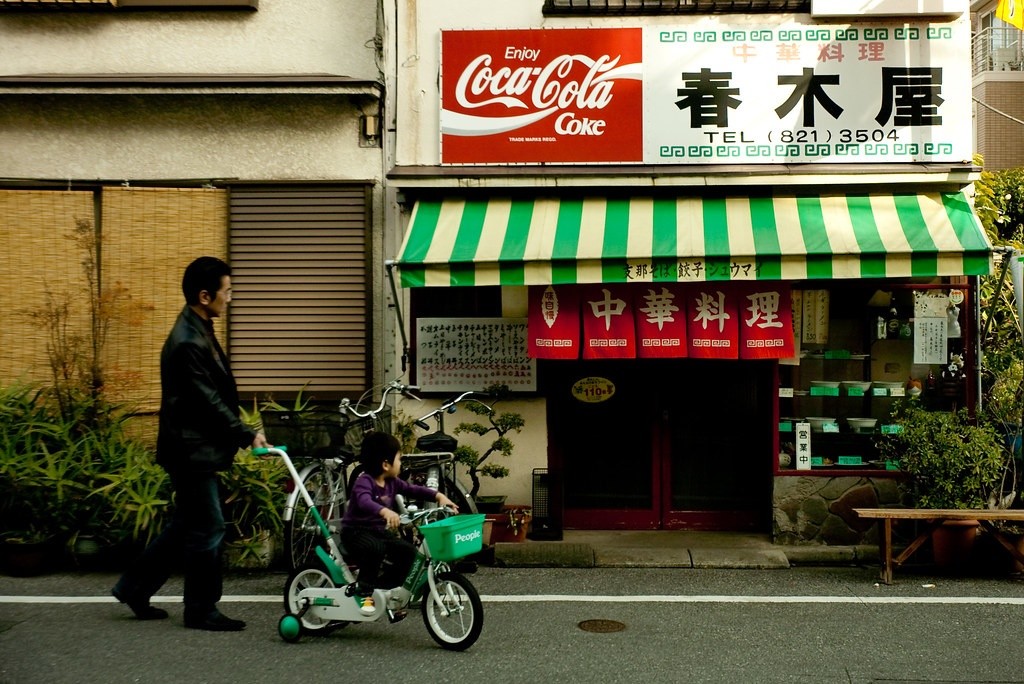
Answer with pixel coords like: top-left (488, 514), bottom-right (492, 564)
top-left (215, 290), bottom-right (233, 301)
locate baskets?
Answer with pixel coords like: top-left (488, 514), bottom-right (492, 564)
top-left (323, 402), bottom-right (392, 457)
top-left (261, 409), bottom-right (351, 459)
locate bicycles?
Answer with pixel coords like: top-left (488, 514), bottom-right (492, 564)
top-left (281, 370), bottom-right (492, 593)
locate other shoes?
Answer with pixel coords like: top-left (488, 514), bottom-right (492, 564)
top-left (112, 584), bottom-right (167, 620)
top-left (183, 607), bottom-right (247, 632)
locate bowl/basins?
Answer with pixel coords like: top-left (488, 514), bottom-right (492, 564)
top-left (846, 418), bottom-right (877, 432)
top-left (781, 417), bottom-right (836, 432)
top-left (811, 381), bottom-right (903, 392)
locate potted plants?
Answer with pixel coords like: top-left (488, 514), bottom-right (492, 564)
top-left (870, 395), bottom-right (1005, 575)
top-left (454, 382), bottom-right (525, 512)
top-left (479, 508), bottom-right (532, 546)
top-left (973, 358), bottom-right (1023, 580)
top-left (0, 366), bottom-right (320, 577)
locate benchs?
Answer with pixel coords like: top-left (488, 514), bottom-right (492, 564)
top-left (852, 506), bottom-right (1023, 586)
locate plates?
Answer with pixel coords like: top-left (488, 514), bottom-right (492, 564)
top-left (800, 354), bottom-right (869, 359)
top-left (811, 460), bottom-right (884, 468)
top-left (793, 390), bottom-right (809, 396)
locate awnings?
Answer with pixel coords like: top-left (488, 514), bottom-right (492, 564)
top-left (394, 192), bottom-right (994, 288)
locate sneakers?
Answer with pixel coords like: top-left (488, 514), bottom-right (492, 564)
top-left (359, 595), bottom-right (377, 615)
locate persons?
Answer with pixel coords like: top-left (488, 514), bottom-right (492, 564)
top-left (111, 256), bottom-right (275, 631)
top-left (341, 431), bottom-right (459, 616)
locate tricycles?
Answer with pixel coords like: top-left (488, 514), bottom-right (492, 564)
top-left (252, 445), bottom-right (484, 652)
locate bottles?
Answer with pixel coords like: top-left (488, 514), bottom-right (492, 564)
top-left (925, 370), bottom-right (939, 395)
